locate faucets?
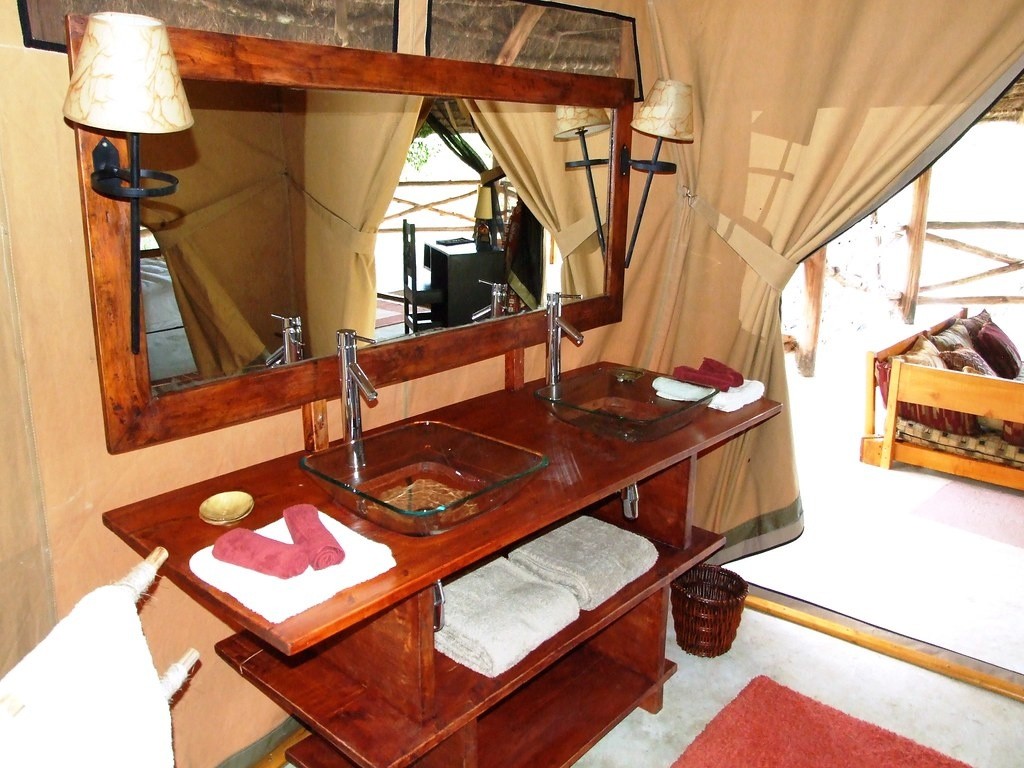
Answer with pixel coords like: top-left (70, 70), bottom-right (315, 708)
top-left (331, 328), bottom-right (380, 441)
top-left (545, 290), bottom-right (586, 385)
top-left (260, 312), bottom-right (305, 368)
top-left (468, 277), bottom-right (511, 320)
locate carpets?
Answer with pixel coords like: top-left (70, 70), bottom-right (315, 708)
top-left (375, 294), bottom-right (422, 333)
top-left (670, 675), bottom-right (974, 767)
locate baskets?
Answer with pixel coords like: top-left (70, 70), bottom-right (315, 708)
top-left (670, 563), bottom-right (749, 658)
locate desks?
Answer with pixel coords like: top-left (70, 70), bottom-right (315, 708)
top-left (422, 236), bottom-right (505, 330)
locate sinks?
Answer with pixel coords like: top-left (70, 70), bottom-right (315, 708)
top-left (531, 365), bottom-right (722, 444)
top-left (295, 417), bottom-right (549, 540)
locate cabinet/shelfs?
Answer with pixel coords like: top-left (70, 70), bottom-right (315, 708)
top-left (98, 360), bottom-right (784, 768)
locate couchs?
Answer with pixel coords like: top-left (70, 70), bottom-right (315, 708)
top-left (858, 305), bottom-right (1024, 487)
top-left (862, 368), bottom-right (1023, 494)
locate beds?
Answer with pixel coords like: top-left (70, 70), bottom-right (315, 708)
top-left (140, 257), bottom-right (200, 385)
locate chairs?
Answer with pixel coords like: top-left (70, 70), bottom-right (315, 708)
top-left (402, 219), bottom-right (436, 336)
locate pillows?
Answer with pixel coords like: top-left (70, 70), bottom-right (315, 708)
top-left (876, 309), bottom-right (1024, 447)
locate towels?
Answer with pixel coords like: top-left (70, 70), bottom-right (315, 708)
top-left (211, 525), bottom-right (307, 580)
top-left (189, 506), bottom-right (398, 623)
top-left (698, 356), bottom-right (744, 387)
top-left (673, 362), bottom-right (730, 393)
top-left (652, 377), bottom-right (766, 414)
top-left (432, 555), bottom-right (583, 679)
top-left (1, 566), bottom-right (177, 766)
top-left (280, 503), bottom-right (348, 570)
top-left (507, 512), bottom-right (661, 609)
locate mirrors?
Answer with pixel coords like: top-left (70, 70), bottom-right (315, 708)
top-left (63, 11), bottom-right (637, 456)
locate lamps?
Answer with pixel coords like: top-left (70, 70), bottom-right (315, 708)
top-left (622, 76), bottom-right (697, 269)
top-left (62, 12), bottom-right (196, 358)
top-left (551, 103), bottom-right (612, 267)
top-left (474, 186), bottom-right (493, 250)
